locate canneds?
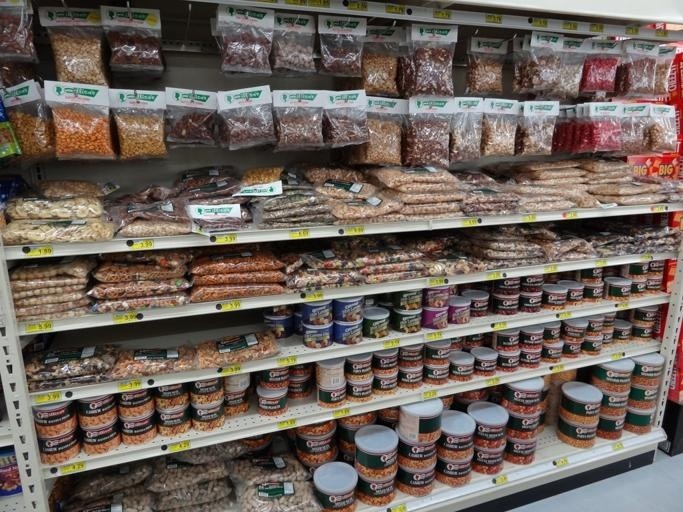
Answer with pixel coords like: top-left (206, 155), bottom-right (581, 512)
top-left (262, 312), bottom-right (291, 338)
top-left (291, 285), bottom-right (471, 349)
top-left (556, 415), bottom-right (597, 450)
top-left (462, 290), bottom-right (490, 318)
top-left (290, 330), bottom-right (498, 407)
top-left (559, 382), bottom-right (600, 428)
top-left (291, 369), bottom-right (576, 512)
top-left (492, 260), bottom-right (665, 372)
top-left (592, 351), bottom-right (665, 441)
top-left (32, 367), bottom-right (289, 462)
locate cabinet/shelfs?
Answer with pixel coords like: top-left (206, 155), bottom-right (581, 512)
top-left (0, 0), bottom-right (683, 512)
